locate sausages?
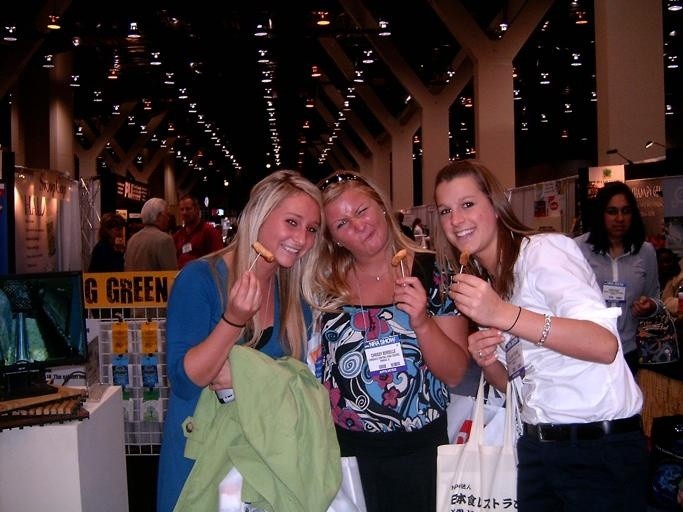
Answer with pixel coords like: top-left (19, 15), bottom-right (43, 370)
top-left (460, 251), bottom-right (470, 265)
top-left (253, 242), bottom-right (274, 261)
top-left (392, 249), bottom-right (407, 266)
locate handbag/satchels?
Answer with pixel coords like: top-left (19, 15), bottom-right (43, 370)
top-left (634, 295), bottom-right (681, 367)
top-left (436, 360), bottom-right (519, 512)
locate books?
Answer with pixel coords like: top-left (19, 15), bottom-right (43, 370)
top-left (0, 384), bottom-right (96, 435)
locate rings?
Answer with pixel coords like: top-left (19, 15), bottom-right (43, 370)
top-left (477, 349), bottom-right (485, 360)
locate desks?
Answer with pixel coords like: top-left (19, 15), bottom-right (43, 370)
top-left (1, 383), bottom-right (130, 511)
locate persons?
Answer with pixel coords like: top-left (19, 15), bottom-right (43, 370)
top-left (154, 170), bottom-right (328, 512)
top-left (570, 179), bottom-right (661, 383)
top-left (395, 212), bottom-right (416, 242)
top-left (300, 168), bottom-right (475, 512)
top-left (85, 189), bottom-right (224, 319)
top-left (430, 156), bottom-right (659, 512)
top-left (411, 218), bottom-right (426, 247)
top-left (423, 224), bottom-right (428, 236)
top-left (216, 223), bottom-right (238, 246)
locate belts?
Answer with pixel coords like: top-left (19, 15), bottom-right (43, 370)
top-left (523, 415), bottom-right (643, 445)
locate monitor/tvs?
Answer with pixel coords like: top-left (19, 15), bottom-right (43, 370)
top-left (0, 270), bottom-right (88, 402)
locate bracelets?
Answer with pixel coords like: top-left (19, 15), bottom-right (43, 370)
top-left (410, 313), bottom-right (431, 331)
top-left (535, 313), bottom-right (553, 348)
top-left (503, 305), bottom-right (523, 333)
top-left (219, 313), bottom-right (246, 332)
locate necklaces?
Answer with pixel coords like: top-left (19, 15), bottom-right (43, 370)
top-left (357, 265), bottom-right (391, 282)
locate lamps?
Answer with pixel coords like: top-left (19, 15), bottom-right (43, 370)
top-left (606, 142), bottom-right (672, 166)
top-left (665, 2), bottom-right (681, 119)
top-left (332, 17), bottom-right (393, 139)
top-left (298, 6), bottom-right (331, 166)
top-left (4, 13), bottom-right (123, 171)
top-left (388, 2), bottom-right (598, 168)
top-left (200, 173), bottom-right (227, 186)
top-left (126, 13), bottom-right (243, 170)
top-left (253, 20), bottom-right (283, 171)
